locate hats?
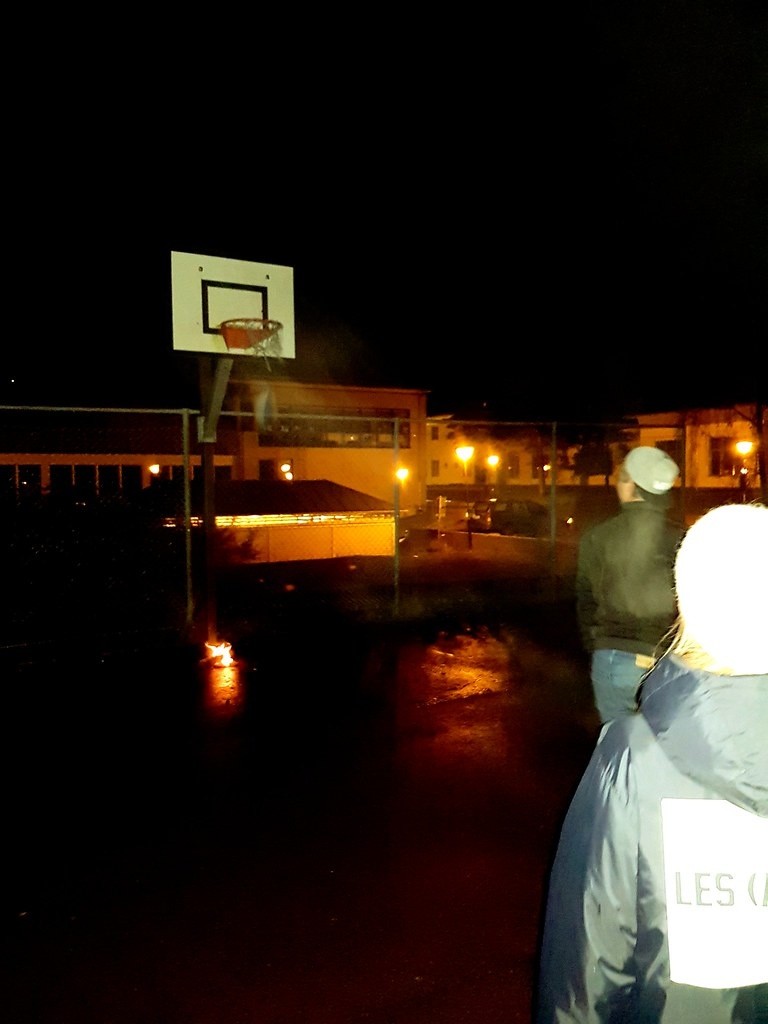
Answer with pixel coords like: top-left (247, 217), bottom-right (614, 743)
top-left (623, 446), bottom-right (680, 509)
top-left (674, 505), bottom-right (768, 673)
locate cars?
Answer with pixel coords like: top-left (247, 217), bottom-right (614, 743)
top-left (467, 496), bottom-right (572, 539)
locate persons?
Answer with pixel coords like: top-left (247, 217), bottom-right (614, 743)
top-left (574, 443), bottom-right (696, 723)
top-left (540, 505), bottom-right (767, 1024)
